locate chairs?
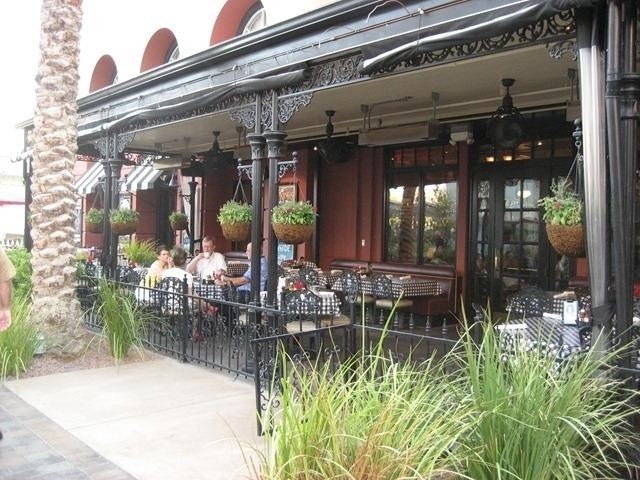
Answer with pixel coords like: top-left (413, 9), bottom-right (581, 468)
top-left (506, 280), bottom-right (640, 382)
top-left (119, 252), bottom-right (416, 351)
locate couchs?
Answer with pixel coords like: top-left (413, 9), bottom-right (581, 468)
top-left (329, 256), bottom-right (458, 320)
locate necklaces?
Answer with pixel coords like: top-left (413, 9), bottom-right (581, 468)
top-left (160, 262), bottom-right (167, 269)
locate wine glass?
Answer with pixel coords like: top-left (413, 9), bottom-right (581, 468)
top-left (96, 266), bottom-right (104, 277)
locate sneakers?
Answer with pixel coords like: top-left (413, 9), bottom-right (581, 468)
top-left (192, 334), bottom-right (204, 343)
top-left (206, 305), bottom-right (220, 316)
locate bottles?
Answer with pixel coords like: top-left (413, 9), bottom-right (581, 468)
top-left (578, 309), bottom-right (589, 322)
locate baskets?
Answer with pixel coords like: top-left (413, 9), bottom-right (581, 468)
top-left (270, 222), bottom-right (315, 245)
top-left (221, 222), bottom-right (252, 243)
top-left (88, 222), bottom-right (104, 233)
top-left (544, 223), bottom-right (587, 260)
top-left (171, 222), bottom-right (189, 231)
top-left (111, 220), bottom-right (138, 236)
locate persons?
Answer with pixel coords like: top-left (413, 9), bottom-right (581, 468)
top-left (0, 248), bottom-right (18, 439)
top-left (423, 229), bottom-right (570, 308)
top-left (183, 235), bottom-right (228, 280)
top-left (136, 243), bottom-right (175, 301)
top-left (159, 249), bottom-right (219, 344)
top-left (213, 240), bottom-right (270, 339)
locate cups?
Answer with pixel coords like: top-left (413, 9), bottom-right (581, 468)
top-left (203, 251), bottom-right (210, 258)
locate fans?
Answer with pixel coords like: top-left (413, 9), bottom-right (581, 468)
top-left (486, 77), bottom-right (527, 152)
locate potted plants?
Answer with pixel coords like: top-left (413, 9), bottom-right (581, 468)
top-left (168, 214), bottom-right (189, 230)
top-left (217, 199), bottom-right (318, 245)
top-left (538, 177), bottom-right (587, 258)
top-left (86, 208), bottom-right (139, 235)
